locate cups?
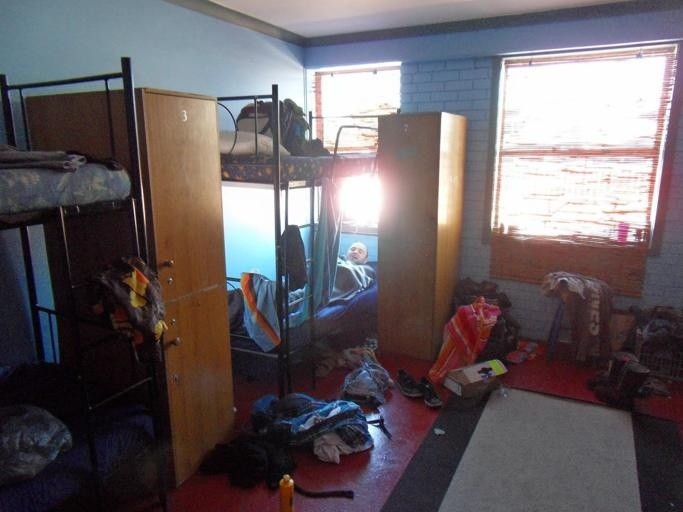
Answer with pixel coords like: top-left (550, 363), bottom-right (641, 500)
top-left (506, 351), bottom-right (523, 378)
top-left (616, 223), bottom-right (628, 241)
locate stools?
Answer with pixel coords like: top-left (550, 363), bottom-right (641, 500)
top-left (546, 300), bottom-right (601, 368)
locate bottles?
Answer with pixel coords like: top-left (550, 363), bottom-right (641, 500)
top-left (494, 378), bottom-right (510, 400)
top-left (279, 474), bottom-right (294, 512)
top-left (639, 228), bottom-right (647, 247)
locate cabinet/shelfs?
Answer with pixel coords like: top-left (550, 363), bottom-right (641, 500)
top-left (378, 111), bottom-right (468, 363)
top-left (24, 88), bottom-right (234, 488)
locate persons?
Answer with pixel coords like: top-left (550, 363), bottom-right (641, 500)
top-left (288, 242), bottom-right (376, 312)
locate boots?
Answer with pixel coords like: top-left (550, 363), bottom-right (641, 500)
top-left (586, 351), bottom-right (650, 409)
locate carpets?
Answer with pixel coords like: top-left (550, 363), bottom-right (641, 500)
top-left (380, 388), bottom-right (683, 511)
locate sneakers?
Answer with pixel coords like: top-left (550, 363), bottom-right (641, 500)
top-left (420, 377), bottom-right (442, 407)
top-left (396, 368), bottom-right (422, 398)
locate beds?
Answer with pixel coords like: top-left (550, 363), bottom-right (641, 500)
top-left (1, 58), bottom-right (168, 511)
top-left (218, 84), bottom-right (401, 400)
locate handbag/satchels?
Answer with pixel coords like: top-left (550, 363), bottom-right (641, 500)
top-left (264, 392), bottom-right (319, 418)
top-left (237, 99), bottom-right (295, 144)
top-left (227, 281), bottom-right (244, 330)
